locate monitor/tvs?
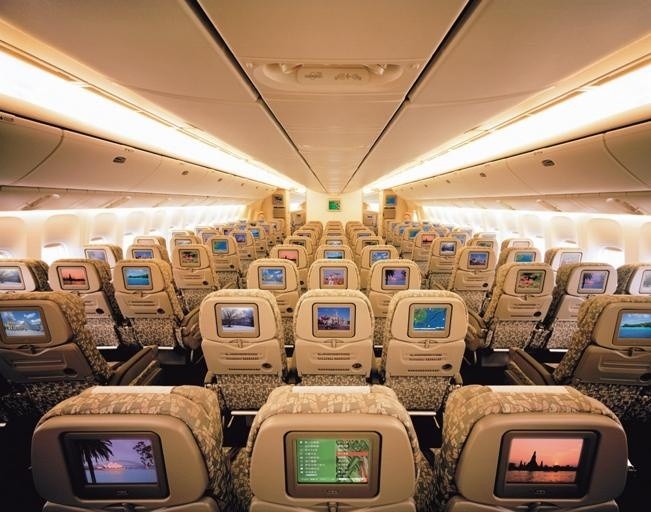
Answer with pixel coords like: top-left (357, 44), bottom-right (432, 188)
top-left (61, 432), bottom-right (169, 499)
top-left (320, 266), bottom-right (348, 289)
top-left (172, 232), bottom-right (185, 237)
top-left (175, 239), bottom-right (191, 246)
top-left (312, 303), bottom-right (355, 338)
top-left (362, 240), bottom-right (378, 249)
top-left (408, 303), bottom-right (451, 338)
top-left (202, 233), bottom-right (216, 245)
top-left (494, 430), bottom-right (598, 499)
top-left (284, 430), bottom-right (381, 499)
top-left (224, 229), bottom-right (230, 235)
top-left (639, 270), bottom-right (651, 294)
top-left (433, 224), bottom-right (452, 238)
top-left (249, 223), bottom-right (256, 228)
top-left (249, 230), bottom-right (259, 238)
top-left (258, 221), bottom-right (264, 224)
top-left (512, 241), bottom-right (530, 249)
top-left (258, 267), bottom-right (286, 290)
top-left (612, 309), bottom-right (651, 346)
top-left (240, 220), bottom-right (246, 225)
top-left (421, 234), bottom-right (435, 246)
top-left (324, 250), bottom-right (345, 260)
top-left (122, 266), bottom-right (153, 290)
top-left (399, 226), bottom-right (407, 234)
top-left (439, 241), bottom-right (456, 255)
top-left (467, 252), bottom-right (489, 269)
top-left (382, 267), bottom-right (409, 289)
top-left (179, 250), bottom-right (201, 267)
top-left (196, 228), bottom-right (203, 234)
top-left (369, 250), bottom-right (391, 267)
top-left (215, 303), bottom-right (259, 338)
top-left (0, 266), bottom-right (25, 290)
top-left (477, 241), bottom-right (494, 249)
top-left (514, 252), bottom-right (535, 262)
top-left (328, 233), bottom-right (341, 236)
top-left (386, 195), bottom-right (398, 206)
top-left (357, 233), bottom-right (371, 238)
top-left (404, 221), bottom-right (410, 225)
top-left (515, 269), bottom-right (545, 294)
top-left (412, 223), bottom-right (419, 228)
top-left (392, 223), bottom-right (397, 228)
top-left (132, 249), bottom-right (153, 259)
top-left (452, 235), bottom-right (466, 247)
top-left (57, 266), bottom-right (90, 290)
top-left (238, 226), bottom-right (245, 231)
top-left (0, 306), bottom-right (51, 344)
top-left (278, 249), bottom-right (299, 267)
top-left (137, 240), bottom-right (153, 245)
top-left (233, 233), bottom-right (247, 244)
top-left (289, 240), bottom-right (305, 247)
top-left (560, 252), bottom-right (581, 265)
top-left (422, 225), bottom-right (431, 232)
top-left (86, 250), bottom-right (107, 264)
top-left (409, 230), bottom-right (419, 239)
top-left (326, 240), bottom-right (342, 246)
top-left (261, 226), bottom-right (269, 234)
top-left (212, 239), bottom-right (228, 253)
top-left (297, 233), bottom-right (311, 238)
top-left (578, 270), bottom-right (609, 294)
top-left (271, 224), bottom-right (277, 230)
